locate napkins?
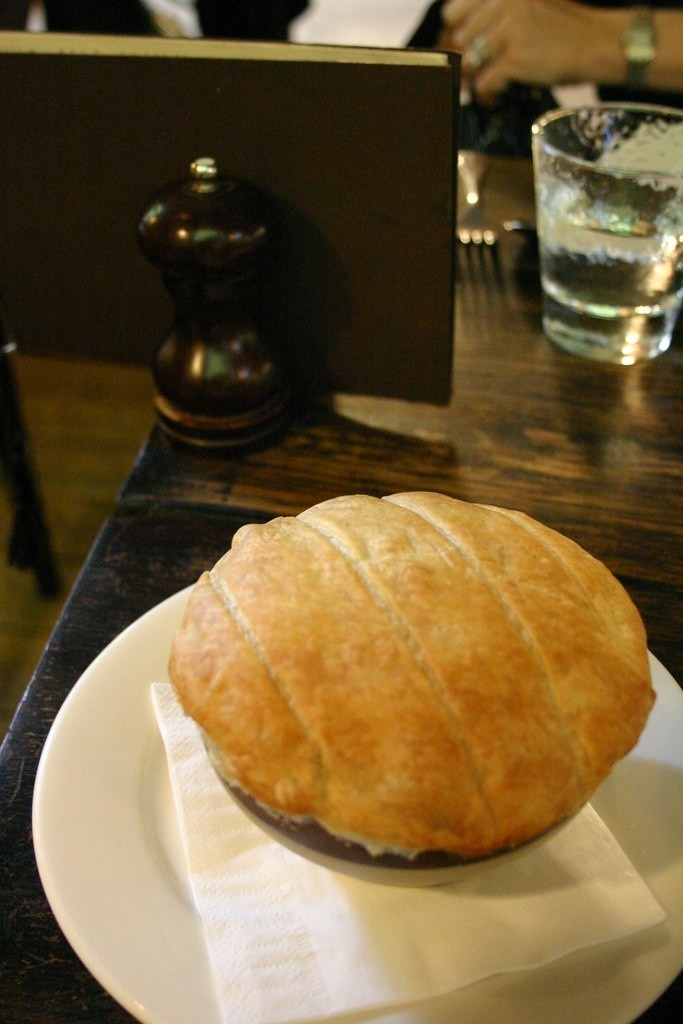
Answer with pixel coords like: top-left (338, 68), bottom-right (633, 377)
top-left (149, 682), bottom-right (664, 1024)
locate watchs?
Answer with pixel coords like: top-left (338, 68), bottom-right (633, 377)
top-left (621, 3), bottom-right (659, 95)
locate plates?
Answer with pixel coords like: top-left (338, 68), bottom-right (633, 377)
top-left (30, 577), bottom-right (683, 1024)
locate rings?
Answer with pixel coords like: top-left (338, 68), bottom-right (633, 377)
top-left (462, 36), bottom-right (494, 68)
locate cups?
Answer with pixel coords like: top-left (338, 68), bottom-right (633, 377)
top-left (531, 100), bottom-right (683, 365)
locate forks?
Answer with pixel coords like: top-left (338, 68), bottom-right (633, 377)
top-left (457, 146), bottom-right (501, 246)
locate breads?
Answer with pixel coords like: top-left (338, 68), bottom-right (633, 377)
top-left (166, 490), bottom-right (657, 862)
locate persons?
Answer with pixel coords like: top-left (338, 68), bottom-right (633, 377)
top-left (443, 0), bottom-right (683, 108)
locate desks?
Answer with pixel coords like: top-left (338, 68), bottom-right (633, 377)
top-left (0, 135), bottom-right (683, 1024)
